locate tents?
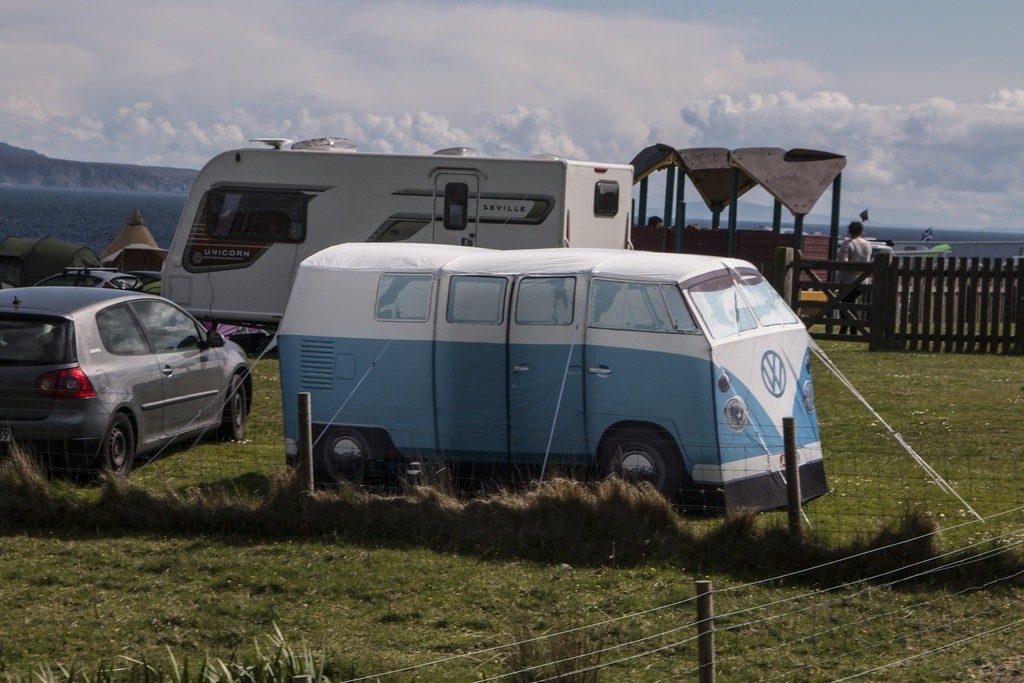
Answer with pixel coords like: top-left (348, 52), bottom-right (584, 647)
top-left (0, 209), bottom-right (166, 287)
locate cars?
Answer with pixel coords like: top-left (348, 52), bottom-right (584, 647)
top-left (124, 271), bottom-right (162, 296)
top-left (32, 267), bottom-right (144, 293)
top-left (0, 286), bottom-right (253, 477)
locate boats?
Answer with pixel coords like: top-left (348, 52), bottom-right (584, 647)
top-left (890, 240), bottom-right (955, 265)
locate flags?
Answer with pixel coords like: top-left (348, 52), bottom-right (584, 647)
top-left (921, 227), bottom-right (933, 242)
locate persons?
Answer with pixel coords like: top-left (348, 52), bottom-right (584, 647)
top-left (647, 216), bottom-right (663, 227)
top-left (687, 224), bottom-right (700, 230)
top-left (838, 221), bottom-right (872, 335)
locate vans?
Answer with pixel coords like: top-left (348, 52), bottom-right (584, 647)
top-left (278, 243), bottom-right (831, 520)
